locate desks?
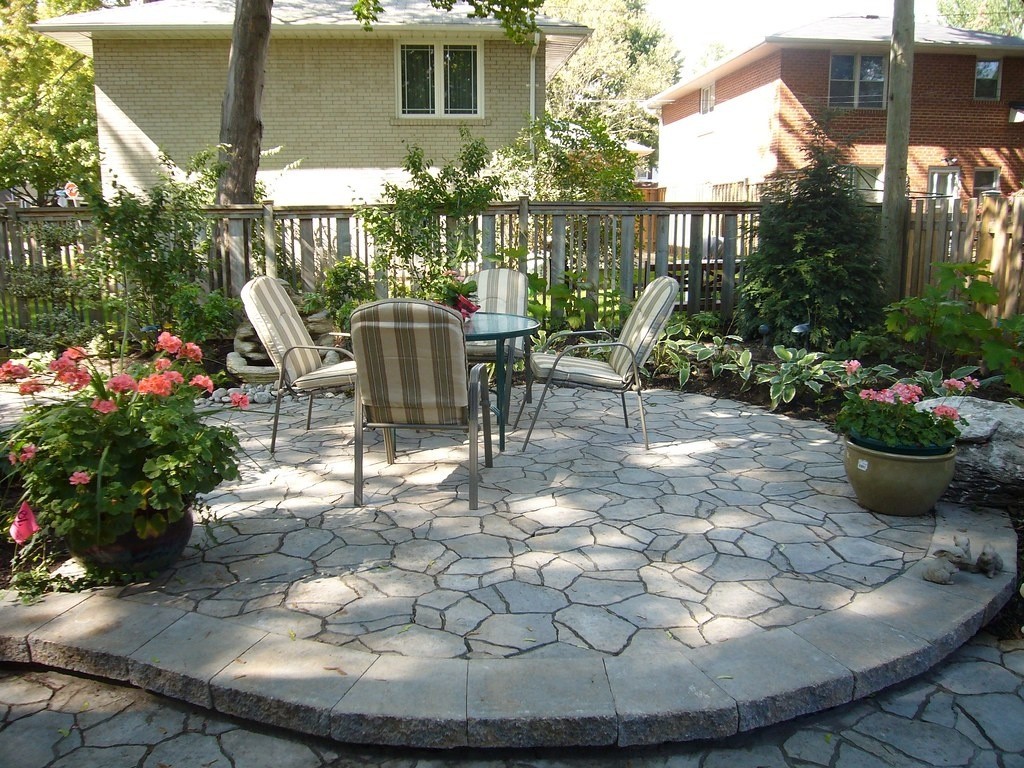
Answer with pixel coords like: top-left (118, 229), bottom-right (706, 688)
top-left (463, 312), bottom-right (539, 451)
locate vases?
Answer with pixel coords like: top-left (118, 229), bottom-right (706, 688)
top-left (70, 492), bottom-right (196, 570)
top-left (843, 429), bottom-right (959, 517)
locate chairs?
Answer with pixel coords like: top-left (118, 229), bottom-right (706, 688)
top-left (240, 276), bottom-right (375, 453)
top-left (512, 275), bottom-right (680, 452)
top-left (348, 299), bottom-right (493, 509)
top-left (458, 267), bottom-right (532, 426)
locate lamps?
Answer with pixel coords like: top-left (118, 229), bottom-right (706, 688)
top-left (141, 325), bottom-right (163, 337)
top-left (791, 324), bottom-right (810, 351)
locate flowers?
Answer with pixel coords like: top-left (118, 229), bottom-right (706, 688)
top-left (429, 270), bottom-right (478, 310)
top-left (832, 357), bottom-right (979, 447)
top-left (0, 325), bottom-right (295, 586)
